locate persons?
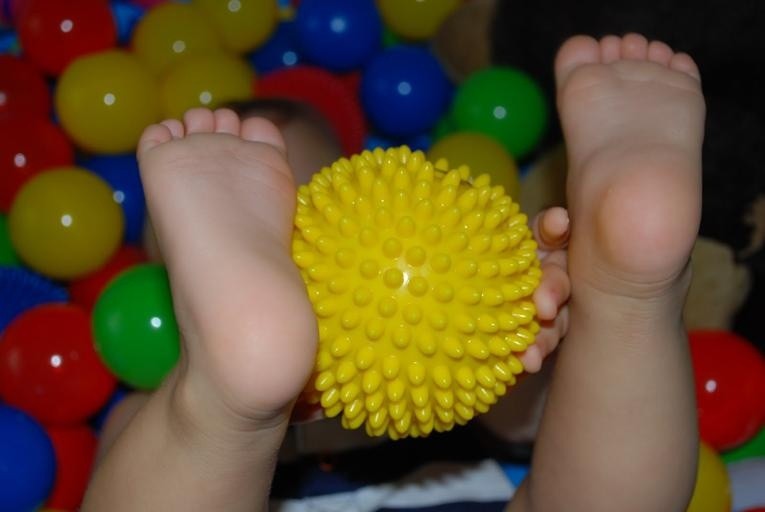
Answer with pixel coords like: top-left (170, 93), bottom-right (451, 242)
top-left (79, 35), bottom-right (706, 512)
top-left (491, 0), bottom-right (765, 353)
